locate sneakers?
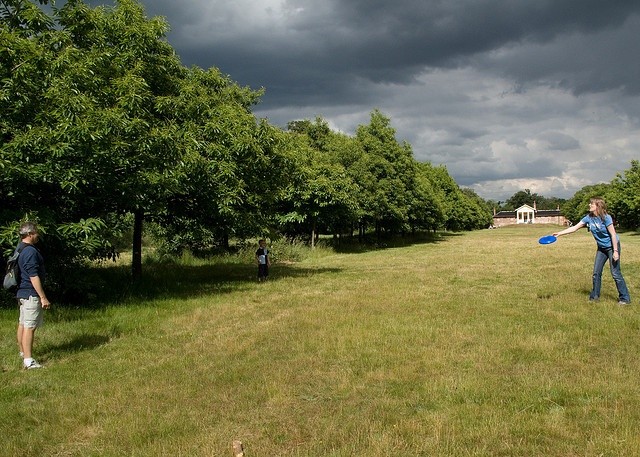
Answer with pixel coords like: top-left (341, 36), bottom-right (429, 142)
top-left (23, 357), bottom-right (42, 369)
top-left (18, 351), bottom-right (23, 357)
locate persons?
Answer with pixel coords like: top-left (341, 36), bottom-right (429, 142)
top-left (552, 196), bottom-right (631, 305)
top-left (16, 223), bottom-right (51, 370)
top-left (256, 240), bottom-right (271, 282)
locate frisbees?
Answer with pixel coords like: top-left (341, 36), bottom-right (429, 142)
top-left (538, 236), bottom-right (556, 244)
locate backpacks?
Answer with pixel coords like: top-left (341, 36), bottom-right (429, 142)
top-left (2, 242), bottom-right (31, 293)
